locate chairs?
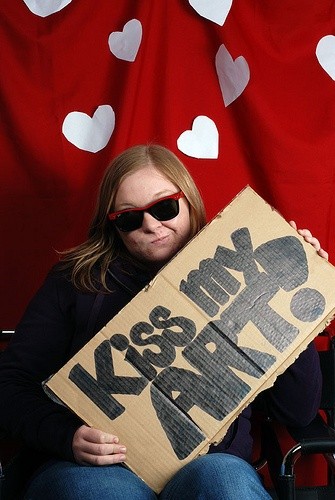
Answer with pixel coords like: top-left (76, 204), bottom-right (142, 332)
top-left (252, 330), bottom-right (334, 500)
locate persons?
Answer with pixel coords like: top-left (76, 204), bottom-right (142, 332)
top-left (1, 145), bottom-right (322, 500)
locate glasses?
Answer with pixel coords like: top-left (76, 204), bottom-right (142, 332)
top-left (109, 191), bottom-right (185, 235)
